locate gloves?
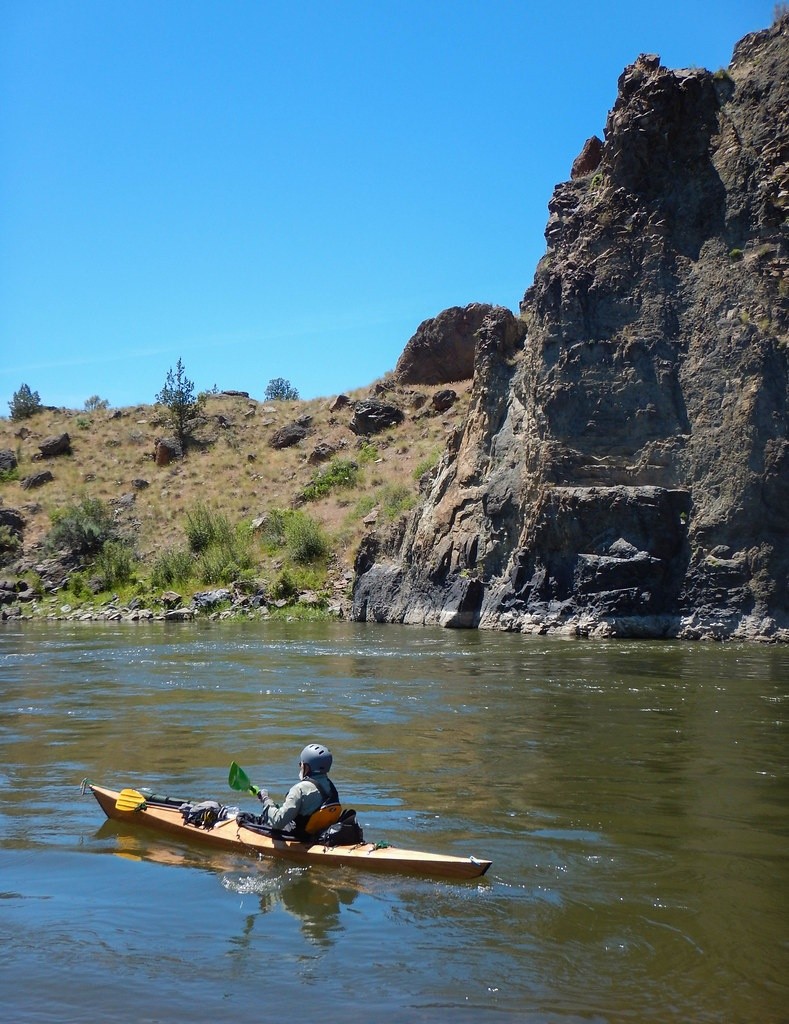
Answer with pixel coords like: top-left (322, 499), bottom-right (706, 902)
top-left (258, 789), bottom-right (268, 800)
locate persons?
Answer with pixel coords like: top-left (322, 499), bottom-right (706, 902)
top-left (237, 742), bottom-right (340, 840)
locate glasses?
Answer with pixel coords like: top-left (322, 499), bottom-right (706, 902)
top-left (298, 761), bottom-right (306, 767)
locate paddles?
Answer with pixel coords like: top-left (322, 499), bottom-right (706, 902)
top-left (114, 787), bottom-right (180, 813)
top-left (226, 760), bottom-right (282, 810)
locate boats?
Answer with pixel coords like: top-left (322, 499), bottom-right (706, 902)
top-left (81, 777), bottom-right (494, 882)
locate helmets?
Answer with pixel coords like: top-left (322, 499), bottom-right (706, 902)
top-left (299, 744), bottom-right (333, 772)
